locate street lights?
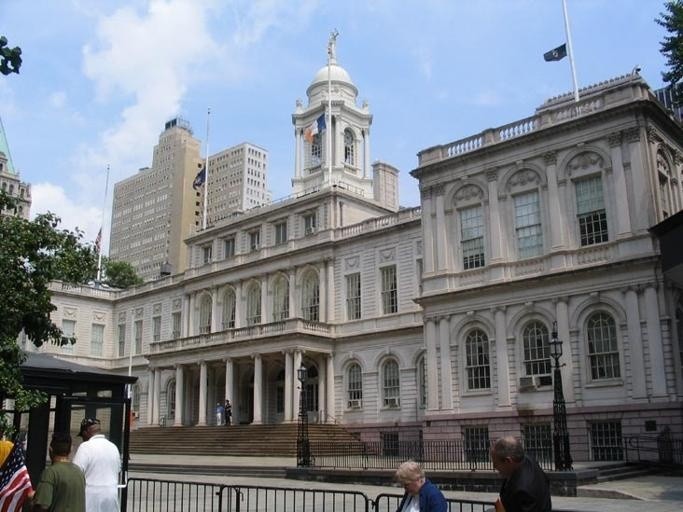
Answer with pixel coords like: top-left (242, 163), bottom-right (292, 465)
top-left (548, 319), bottom-right (575, 472)
top-left (294, 360), bottom-right (313, 468)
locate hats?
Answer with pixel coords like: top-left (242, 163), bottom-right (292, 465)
top-left (77, 417), bottom-right (101, 436)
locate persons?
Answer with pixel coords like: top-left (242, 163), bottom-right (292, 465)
top-left (0, 421), bottom-right (34, 512)
top-left (392, 458), bottom-right (447, 512)
top-left (70, 417), bottom-right (122, 512)
top-left (223, 399), bottom-right (232, 426)
top-left (486, 435), bottom-right (552, 511)
top-left (30, 430), bottom-right (86, 512)
top-left (214, 401), bottom-right (224, 426)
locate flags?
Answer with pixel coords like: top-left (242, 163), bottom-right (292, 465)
top-left (303, 112), bottom-right (326, 145)
top-left (542, 42), bottom-right (567, 63)
top-left (191, 167), bottom-right (204, 190)
top-left (92, 226), bottom-right (101, 256)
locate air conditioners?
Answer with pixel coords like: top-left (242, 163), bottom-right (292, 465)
top-left (351, 399), bottom-right (361, 408)
top-left (388, 397), bottom-right (399, 407)
top-left (518, 376), bottom-right (540, 388)
